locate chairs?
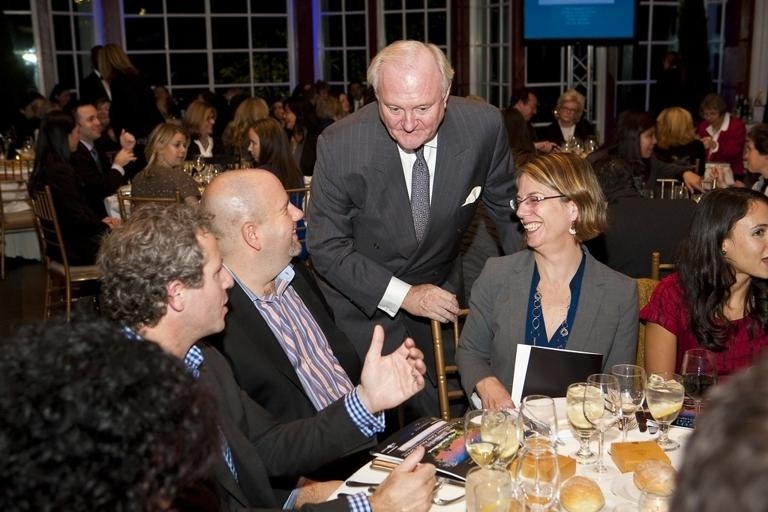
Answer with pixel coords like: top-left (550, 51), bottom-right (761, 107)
top-left (1, 134), bottom-right (768, 511)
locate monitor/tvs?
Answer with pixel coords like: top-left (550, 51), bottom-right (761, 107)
top-left (519, 0), bottom-right (640, 44)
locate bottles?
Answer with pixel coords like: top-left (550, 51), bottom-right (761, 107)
top-left (732, 94), bottom-right (753, 125)
top-left (753, 89), bottom-right (766, 123)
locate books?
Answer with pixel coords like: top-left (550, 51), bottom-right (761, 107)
top-left (511, 345), bottom-right (603, 403)
top-left (370, 416), bottom-right (535, 486)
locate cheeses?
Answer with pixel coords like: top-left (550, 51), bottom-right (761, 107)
top-left (611, 441), bottom-right (671, 474)
top-left (510, 450), bottom-right (577, 490)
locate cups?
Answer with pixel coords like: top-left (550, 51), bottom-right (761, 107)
top-left (560, 137), bottom-right (599, 159)
top-left (673, 185), bottom-right (689, 201)
top-left (182, 154), bottom-right (219, 190)
top-left (641, 189), bottom-right (653, 199)
top-left (460, 395), bottom-right (562, 512)
top-left (638, 488), bottom-right (672, 512)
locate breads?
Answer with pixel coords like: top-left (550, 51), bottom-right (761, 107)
top-left (633, 459), bottom-right (678, 496)
top-left (560, 476), bottom-right (605, 511)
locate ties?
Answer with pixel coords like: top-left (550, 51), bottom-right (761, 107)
top-left (410, 144), bottom-right (432, 248)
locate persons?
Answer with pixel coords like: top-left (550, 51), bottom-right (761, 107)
top-left (1, 319), bottom-right (219, 512)
top-left (656, 51), bottom-right (693, 109)
top-left (2, 81), bottom-right (377, 295)
top-left (457, 153), bottom-right (638, 415)
top-left (206, 169), bottom-right (405, 490)
top-left (503, 89), bottom-right (767, 280)
top-left (639, 185), bottom-right (768, 509)
top-left (85, 40), bottom-right (164, 143)
top-left (97, 202), bottom-right (438, 512)
top-left (306, 38), bottom-right (526, 432)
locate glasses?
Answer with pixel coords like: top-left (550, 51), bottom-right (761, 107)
top-left (509, 194), bottom-right (566, 210)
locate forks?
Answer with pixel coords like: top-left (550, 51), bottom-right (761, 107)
top-left (368, 487), bottom-right (466, 505)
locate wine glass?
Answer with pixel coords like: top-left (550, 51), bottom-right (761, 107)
top-left (564, 363), bottom-right (684, 465)
top-left (682, 347), bottom-right (718, 417)
